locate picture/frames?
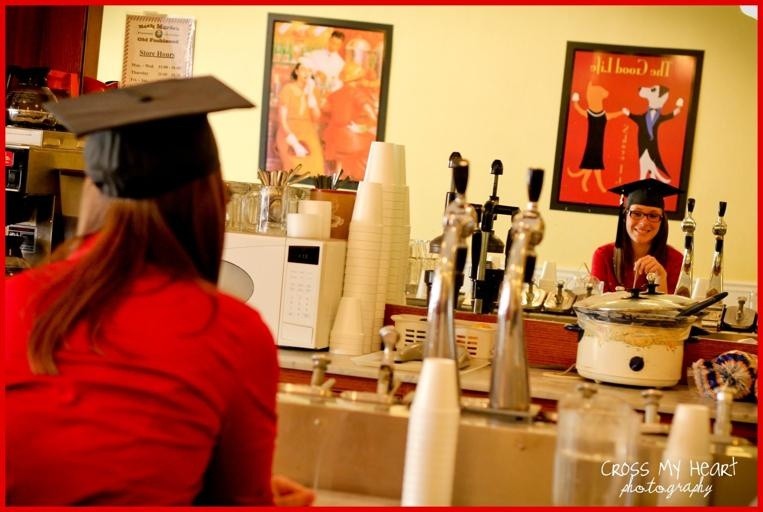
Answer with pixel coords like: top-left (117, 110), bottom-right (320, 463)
top-left (547, 41), bottom-right (705, 222)
top-left (256, 11), bottom-right (393, 190)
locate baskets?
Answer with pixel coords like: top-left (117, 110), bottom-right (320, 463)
top-left (391, 313), bottom-right (499, 361)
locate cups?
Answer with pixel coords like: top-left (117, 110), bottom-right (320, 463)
top-left (550, 384), bottom-right (714, 507)
top-left (692, 278), bottom-right (710, 301)
top-left (536, 261), bottom-right (559, 296)
top-left (398, 237), bottom-right (441, 284)
top-left (398, 355), bottom-right (459, 508)
top-left (223, 177), bottom-right (360, 240)
top-left (328, 140), bottom-right (413, 360)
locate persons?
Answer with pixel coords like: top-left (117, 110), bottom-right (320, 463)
top-left (314, 38), bottom-right (376, 186)
top-left (0, 72), bottom-right (316, 512)
top-left (301, 28), bottom-right (344, 89)
top-left (581, 177), bottom-right (691, 299)
top-left (278, 57), bottom-right (322, 175)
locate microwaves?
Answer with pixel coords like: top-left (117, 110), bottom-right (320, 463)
top-left (216, 229), bottom-right (347, 353)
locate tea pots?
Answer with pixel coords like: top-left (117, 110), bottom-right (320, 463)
top-left (5, 63), bottom-right (60, 128)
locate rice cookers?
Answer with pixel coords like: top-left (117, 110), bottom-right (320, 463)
top-left (682, 305), bottom-right (710, 336)
top-left (564, 288), bottom-right (695, 394)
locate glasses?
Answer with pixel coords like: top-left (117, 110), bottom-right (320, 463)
top-left (626, 209), bottom-right (665, 222)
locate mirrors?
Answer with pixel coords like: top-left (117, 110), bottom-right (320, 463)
top-left (75, 3), bottom-right (759, 429)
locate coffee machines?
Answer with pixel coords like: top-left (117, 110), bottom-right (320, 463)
top-left (4, 128), bottom-right (86, 279)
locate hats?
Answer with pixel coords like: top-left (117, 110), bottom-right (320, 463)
top-left (42, 76), bottom-right (255, 198)
top-left (608, 179), bottom-right (681, 249)
